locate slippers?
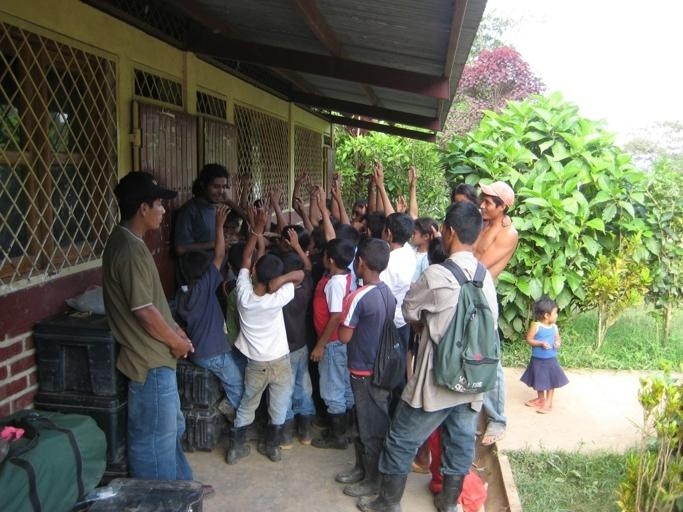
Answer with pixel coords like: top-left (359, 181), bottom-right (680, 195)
top-left (526, 398), bottom-right (552, 414)
top-left (483, 423), bottom-right (504, 446)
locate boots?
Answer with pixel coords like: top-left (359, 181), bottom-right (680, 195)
top-left (434, 476), bottom-right (463, 512)
top-left (226, 413), bottom-right (406, 512)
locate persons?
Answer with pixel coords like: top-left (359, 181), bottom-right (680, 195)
top-left (520, 297), bottom-right (569, 414)
top-left (100, 170), bottom-right (215, 497)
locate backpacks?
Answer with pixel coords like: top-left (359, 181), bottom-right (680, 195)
top-left (433, 259), bottom-right (499, 394)
top-left (370, 288), bottom-right (404, 389)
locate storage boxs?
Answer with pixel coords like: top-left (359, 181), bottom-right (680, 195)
top-left (29, 304), bottom-right (222, 493)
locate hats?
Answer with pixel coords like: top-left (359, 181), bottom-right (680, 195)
top-left (114, 171), bottom-right (177, 199)
top-left (481, 182), bottom-right (514, 206)
